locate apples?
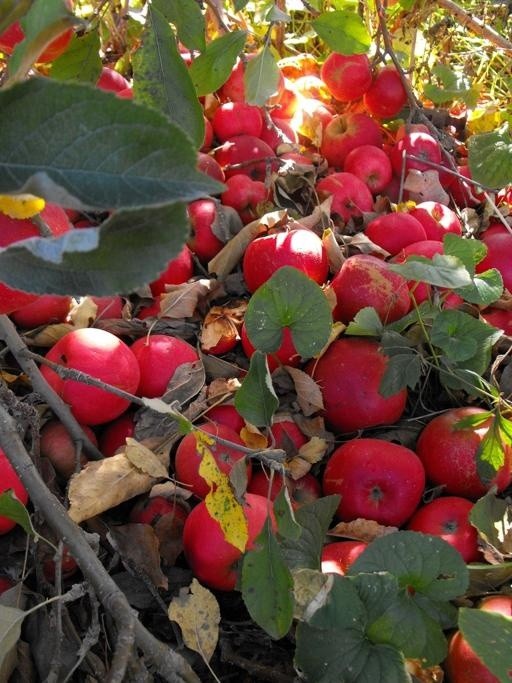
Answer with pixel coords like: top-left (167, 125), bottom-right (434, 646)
top-left (418, 407), bottom-right (512, 496)
top-left (480, 307), bottom-right (511, 336)
top-left (136, 296), bottom-right (203, 322)
top-left (396, 123), bottom-right (430, 145)
top-left (222, 174), bottom-right (273, 226)
top-left (40, 420), bottom-right (100, 482)
top-left (305, 338), bottom-right (408, 432)
top-left (130, 334), bottom-right (200, 398)
top-left (269, 80), bottom-right (299, 119)
top-left (448, 596), bottom-right (512, 683)
top-left (242, 319), bottom-right (302, 371)
top-left (0, 203), bottom-right (75, 326)
top-left (322, 113), bottom-right (383, 167)
top-left (316, 173), bottom-right (372, 221)
top-left (495, 186), bottom-right (512, 215)
top-left (85, 289), bottom-right (123, 318)
top-left (197, 155), bottom-right (226, 182)
top-left (344, 145), bottom-right (393, 193)
top-left (282, 151), bottom-right (314, 167)
top-left (0, 448), bottom-right (27, 537)
top-left (328, 254), bottom-right (411, 325)
top-left (219, 58), bottom-right (285, 108)
top-left (321, 51), bottom-right (374, 103)
top-left (320, 541), bottom-right (369, 576)
top-left (97, 68), bottom-right (128, 96)
top-left (450, 165), bottom-right (487, 207)
top-left (364, 212), bottom-right (427, 256)
top-left (411, 496), bottom-right (479, 562)
top-left (243, 229), bottom-right (330, 293)
top-left (392, 240), bottom-right (461, 306)
top-left (476, 224), bottom-right (512, 295)
top-left (217, 135), bottom-right (280, 183)
top-left (248, 470), bottom-right (317, 519)
top-left (438, 154), bottom-right (457, 190)
top-left (129, 495), bottom-right (186, 525)
top-left (212, 101), bottom-right (262, 141)
top-left (323, 438), bottom-right (426, 526)
top-left (408, 201), bottom-right (463, 243)
top-left (40, 328), bottom-right (141, 425)
top-left (266, 420), bottom-right (307, 455)
top-left (0, 22), bottom-right (75, 62)
top-left (149, 248), bottom-right (194, 292)
top-left (202, 405), bottom-right (245, 435)
top-left (201, 114), bottom-right (212, 151)
top-left (295, 76), bottom-right (332, 107)
top-left (175, 423), bottom-right (252, 502)
top-left (392, 133), bottom-right (442, 178)
top-left (181, 493), bottom-right (277, 588)
top-left (187, 200), bottom-right (226, 256)
top-left (439, 291), bottom-right (465, 309)
top-left (364, 67), bottom-right (408, 119)
top-left (291, 99), bottom-right (338, 147)
top-left (104, 415), bottom-right (136, 458)
